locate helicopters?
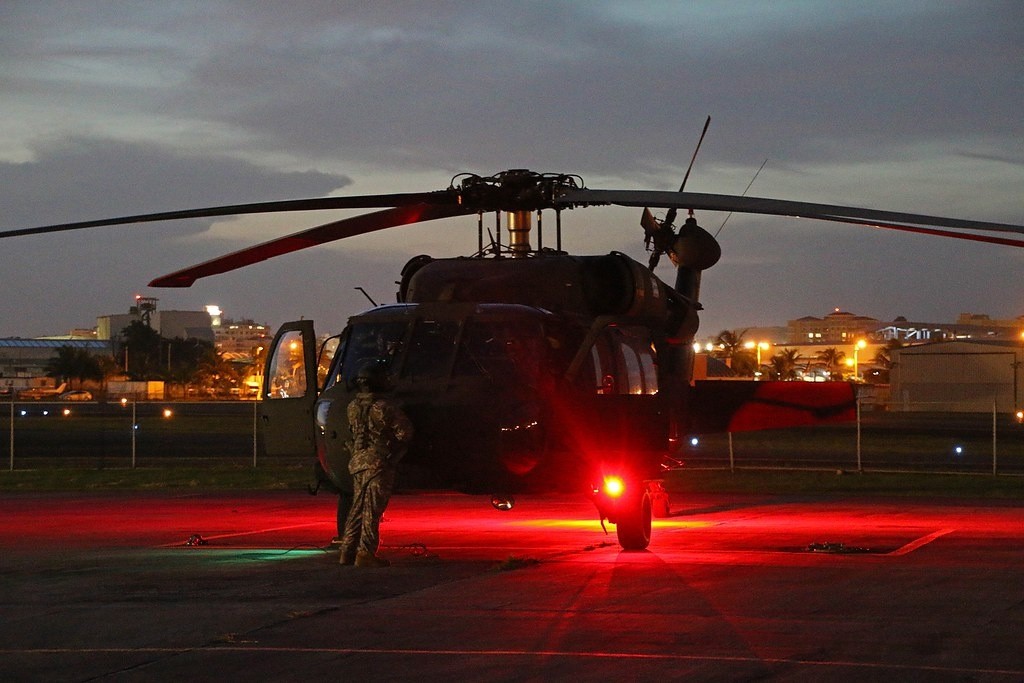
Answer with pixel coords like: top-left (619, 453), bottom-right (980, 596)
top-left (0, 168), bottom-right (1024, 553)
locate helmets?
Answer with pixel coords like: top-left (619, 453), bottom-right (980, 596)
top-left (354, 367), bottom-right (383, 386)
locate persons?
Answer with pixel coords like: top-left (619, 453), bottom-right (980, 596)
top-left (339, 368), bottom-right (415, 569)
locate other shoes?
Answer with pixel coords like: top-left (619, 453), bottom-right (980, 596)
top-left (339, 550), bottom-right (355, 565)
top-left (354, 554), bottom-right (389, 568)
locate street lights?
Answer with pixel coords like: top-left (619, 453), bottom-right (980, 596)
top-left (853, 339), bottom-right (867, 382)
top-left (746, 341), bottom-right (771, 381)
top-left (256, 346), bottom-right (265, 400)
